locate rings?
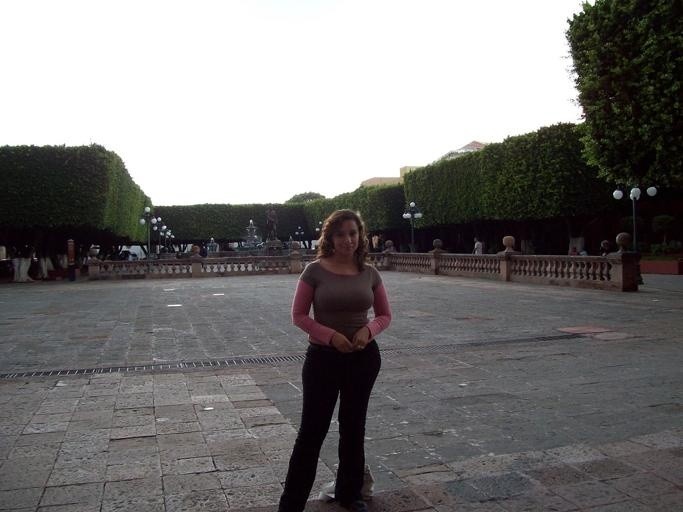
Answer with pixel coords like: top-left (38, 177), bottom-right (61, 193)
top-left (360, 346), bottom-right (364, 348)
top-left (358, 345), bottom-right (360, 348)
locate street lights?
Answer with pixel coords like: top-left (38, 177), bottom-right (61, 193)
top-left (295, 226), bottom-right (304, 248)
top-left (613, 186), bottom-right (656, 252)
top-left (139, 206), bottom-right (174, 258)
top-left (402, 201), bottom-right (422, 253)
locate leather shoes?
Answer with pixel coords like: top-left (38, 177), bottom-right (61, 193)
top-left (345, 499), bottom-right (368, 512)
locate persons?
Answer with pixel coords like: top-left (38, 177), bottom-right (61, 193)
top-left (276, 208), bottom-right (392, 511)
top-left (577, 248), bottom-right (588, 256)
top-left (600, 240), bottom-right (612, 281)
top-left (570, 246), bottom-right (579, 256)
top-left (471, 236), bottom-right (482, 254)
